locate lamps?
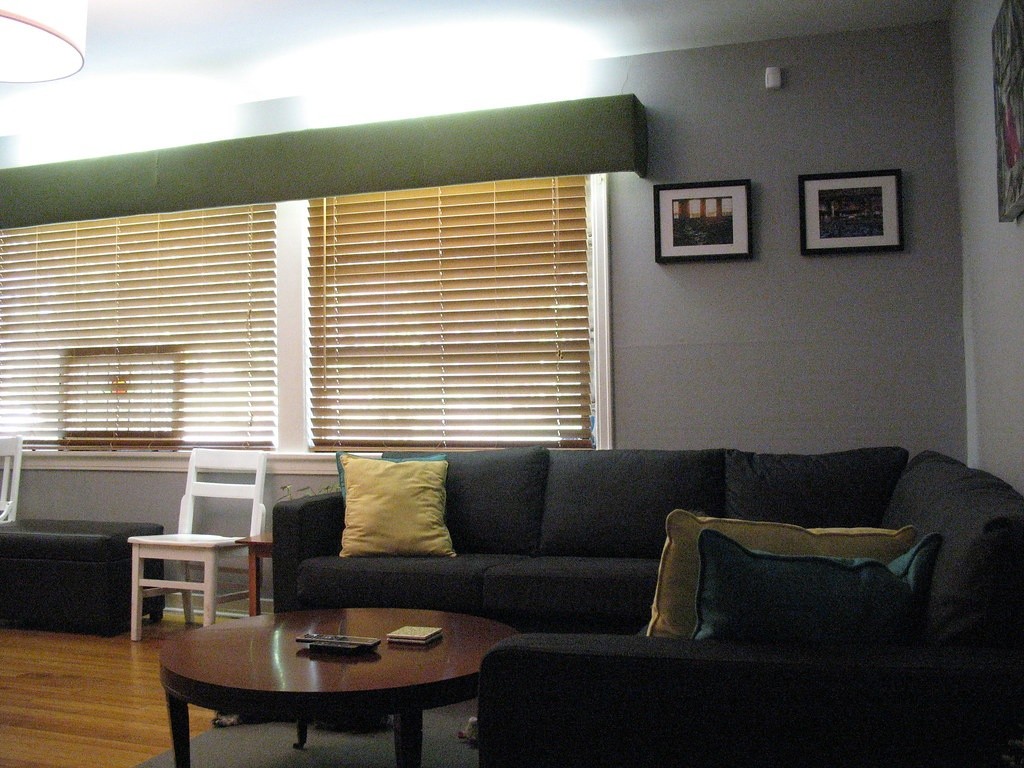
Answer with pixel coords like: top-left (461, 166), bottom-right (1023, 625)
top-left (0, 1), bottom-right (85, 84)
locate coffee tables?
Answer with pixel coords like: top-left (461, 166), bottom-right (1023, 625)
top-left (161, 606), bottom-right (517, 768)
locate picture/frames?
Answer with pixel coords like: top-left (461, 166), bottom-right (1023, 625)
top-left (991, 0), bottom-right (1023, 223)
top-left (654, 179), bottom-right (751, 263)
top-left (60, 347), bottom-right (182, 453)
top-left (798, 169), bottom-right (903, 253)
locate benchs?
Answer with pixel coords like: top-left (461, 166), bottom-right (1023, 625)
top-left (0, 519), bottom-right (163, 634)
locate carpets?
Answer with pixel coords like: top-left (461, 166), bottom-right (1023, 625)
top-left (137, 697), bottom-right (479, 767)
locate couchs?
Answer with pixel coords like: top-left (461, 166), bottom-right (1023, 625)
top-left (274, 448), bottom-right (1024, 768)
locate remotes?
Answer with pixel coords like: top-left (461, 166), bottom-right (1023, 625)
top-left (295, 633), bottom-right (382, 653)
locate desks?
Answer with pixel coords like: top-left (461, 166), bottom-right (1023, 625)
top-left (236, 529), bottom-right (275, 615)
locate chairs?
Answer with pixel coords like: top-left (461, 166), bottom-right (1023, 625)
top-left (125, 448), bottom-right (266, 638)
top-left (1, 438), bottom-right (22, 523)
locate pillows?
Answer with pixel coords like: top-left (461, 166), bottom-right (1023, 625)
top-left (646, 510), bottom-right (916, 643)
top-left (695, 530), bottom-right (940, 648)
top-left (338, 453), bottom-right (456, 559)
